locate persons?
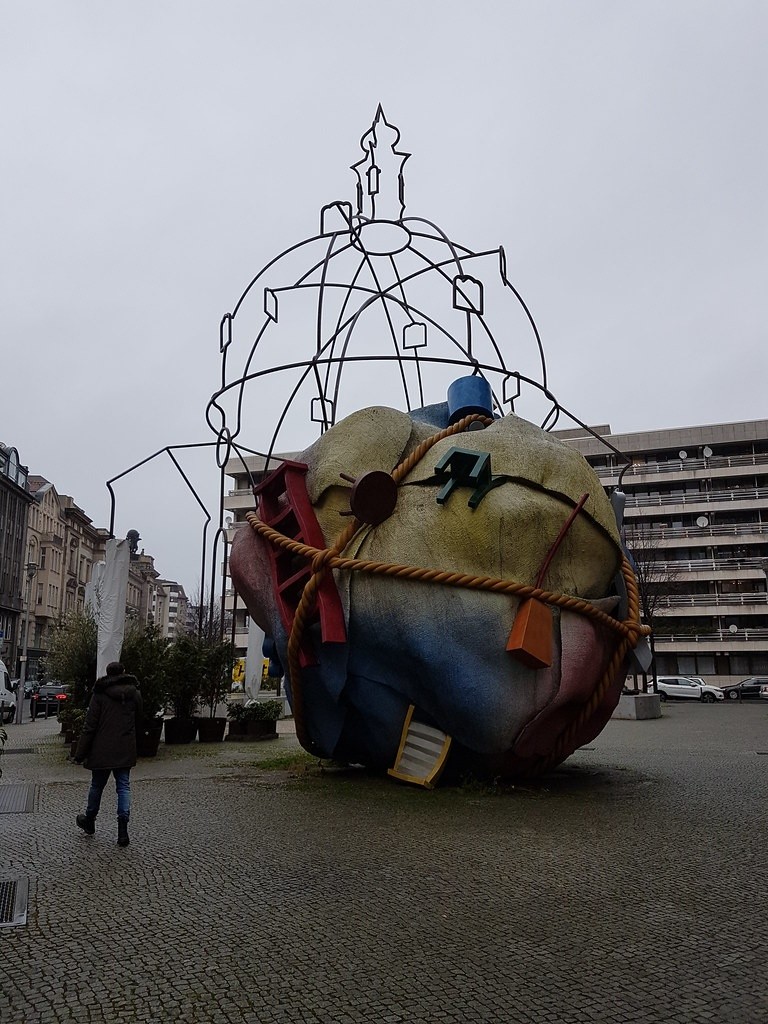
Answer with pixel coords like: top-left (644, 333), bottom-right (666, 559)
top-left (74, 661), bottom-right (145, 846)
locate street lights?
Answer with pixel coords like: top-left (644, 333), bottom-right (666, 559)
top-left (16, 562), bottom-right (39, 725)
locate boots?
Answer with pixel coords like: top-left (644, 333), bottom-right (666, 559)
top-left (76, 808), bottom-right (98, 834)
top-left (118, 821), bottom-right (130, 845)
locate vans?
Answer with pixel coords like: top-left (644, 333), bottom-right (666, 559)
top-left (0, 660), bottom-right (18, 725)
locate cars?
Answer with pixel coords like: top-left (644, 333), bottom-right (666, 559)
top-left (30, 683), bottom-right (69, 717)
top-left (722, 675), bottom-right (768, 702)
top-left (646, 675), bottom-right (723, 703)
